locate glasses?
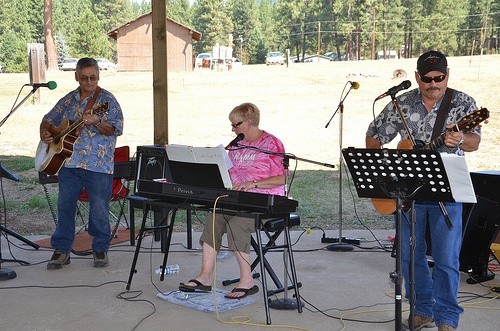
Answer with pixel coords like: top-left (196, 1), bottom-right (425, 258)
top-left (77, 72), bottom-right (97, 82)
top-left (232, 121), bottom-right (244, 128)
top-left (419, 74), bottom-right (446, 83)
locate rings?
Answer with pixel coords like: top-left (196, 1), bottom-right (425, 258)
top-left (241, 187), bottom-right (245, 190)
top-left (85, 119), bottom-right (87, 122)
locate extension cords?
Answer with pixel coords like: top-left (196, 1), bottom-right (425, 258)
top-left (321, 237), bottom-right (361, 245)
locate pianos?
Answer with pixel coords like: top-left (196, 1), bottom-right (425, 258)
top-left (125, 179), bottom-right (304, 325)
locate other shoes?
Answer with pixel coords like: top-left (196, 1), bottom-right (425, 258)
top-left (438, 324), bottom-right (456, 331)
top-left (402, 316), bottom-right (433, 331)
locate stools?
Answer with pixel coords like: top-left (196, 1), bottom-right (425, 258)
top-left (222, 214), bottom-right (300, 290)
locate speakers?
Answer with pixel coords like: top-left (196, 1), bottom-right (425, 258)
top-left (135, 145), bottom-right (173, 200)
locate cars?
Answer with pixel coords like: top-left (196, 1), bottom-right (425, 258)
top-left (264, 52), bottom-right (287, 65)
top-left (95, 58), bottom-right (110, 71)
top-left (61, 58), bottom-right (79, 71)
top-left (196, 54), bottom-right (212, 67)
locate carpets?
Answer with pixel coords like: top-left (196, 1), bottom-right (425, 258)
top-left (21, 226), bottom-right (146, 253)
top-left (156, 288), bottom-right (257, 313)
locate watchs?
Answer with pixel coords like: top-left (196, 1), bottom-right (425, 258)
top-left (253, 180), bottom-right (258, 188)
top-left (94, 118), bottom-right (101, 128)
top-left (457, 134), bottom-right (464, 147)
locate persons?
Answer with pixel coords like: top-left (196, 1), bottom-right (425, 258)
top-left (365, 51), bottom-right (481, 331)
top-left (40, 58), bottom-right (123, 269)
top-left (178, 103), bottom-right (290, 299)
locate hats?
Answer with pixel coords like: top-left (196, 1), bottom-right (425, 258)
top-left (417, 51), bottom-right (447, 75)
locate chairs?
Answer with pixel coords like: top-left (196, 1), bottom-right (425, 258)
top-left (52, 146), bottom-right (130, 242)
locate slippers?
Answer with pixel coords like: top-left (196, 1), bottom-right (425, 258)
top-left (224, 285), bottom-right (259, 299)
top-left (179, 280), bottom-right (212, 292)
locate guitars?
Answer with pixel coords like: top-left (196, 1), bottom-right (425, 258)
top-left (34, 101), bottom-right (110, 175)
top-left (370, 106), bottom-right (490, 216)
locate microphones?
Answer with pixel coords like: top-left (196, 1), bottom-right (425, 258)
top-left (348, 81), bottom-right (360, 90)
top-left (32, 81), bottom-right (57, 90)
top-left (375, 79), bottom-right (412, 100)
top-left (226, 133), bottom-right (245, 148)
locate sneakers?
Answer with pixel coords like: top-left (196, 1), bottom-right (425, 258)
top-left (47, 249), bottom-right (70, 269)
top-left (93, 252), bottom-right (108, 266)
